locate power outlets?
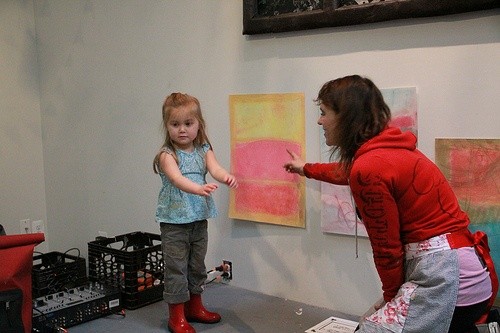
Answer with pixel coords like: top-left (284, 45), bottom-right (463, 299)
top-left (222, 260), bottom-right (233, 281)
top-left (31, 220), bottom-right (44, 233)
top-left (18, 218), bottom-right (31, 234)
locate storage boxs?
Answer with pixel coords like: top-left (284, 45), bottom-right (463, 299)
top-left (32, 251), bottom-right (87, 298)
top-left (87, 231), bottom-right (164, 312)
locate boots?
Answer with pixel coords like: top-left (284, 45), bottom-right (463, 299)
top-left (183, 294), bottom-right (221, 324)
top-left (167, 302), bottom-right (196, 333)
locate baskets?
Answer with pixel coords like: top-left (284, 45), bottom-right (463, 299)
top-left (87, 231), bottom-right (165, 310)
top-left (32, 251), bottom-right (87, 298)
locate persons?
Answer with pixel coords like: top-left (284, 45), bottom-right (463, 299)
top-left (152, 92), bottom-right (239, 333)
top-left (282, 75), bottom-right (500, 333)
